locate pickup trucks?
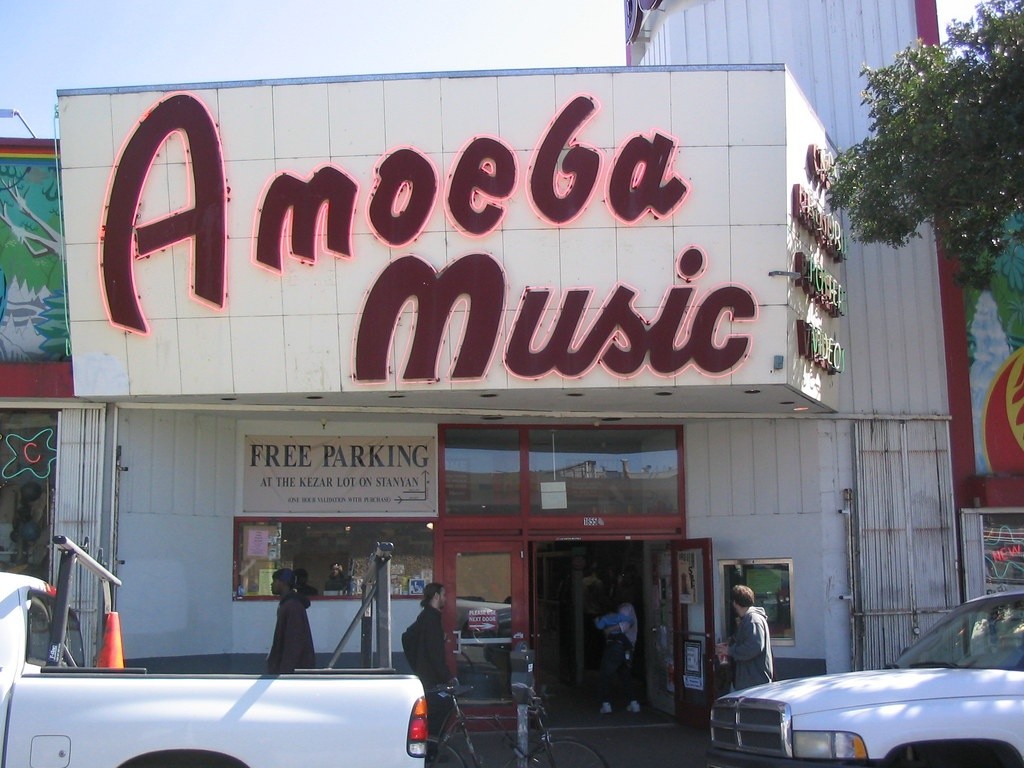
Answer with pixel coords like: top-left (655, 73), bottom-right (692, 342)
top-left (0, 572), bottom-right (429, 768)
top-left (708, 589), bottom-right (1024, 768)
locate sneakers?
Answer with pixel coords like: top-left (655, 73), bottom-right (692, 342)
top-left (600, 702), bottom-right (612, 711)
top-left (627, 700), bottom-right (640, 713)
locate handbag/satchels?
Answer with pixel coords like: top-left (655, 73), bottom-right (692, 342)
top-left (402, 621), bottom-right (420, 673)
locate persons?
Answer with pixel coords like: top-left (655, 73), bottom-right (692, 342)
top-left (267, 567), bottom-right (314, 675)
top-left (714, 585), bottom-right (772, 691)
top-left (593, 599), bottom-right (636, 660)
top-left (596, 592), bottom-right (640, 716)
top-left (325, 562), bottom-right (348, 594)
top-left (401, 583), bottom-right (460, 767)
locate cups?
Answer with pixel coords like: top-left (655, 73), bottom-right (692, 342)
top-left (715, 643), bottom-right (729, 665)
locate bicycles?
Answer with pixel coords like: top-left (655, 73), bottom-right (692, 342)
top-left (423, 678), bottom-right (618, 768)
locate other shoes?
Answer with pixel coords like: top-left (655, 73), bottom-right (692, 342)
top-left (426, 754), bottom-right (447, 764)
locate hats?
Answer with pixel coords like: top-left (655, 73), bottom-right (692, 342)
top-left (273, 568), bottom-right (295, 587)
top-left (330, 563), bottom-right (339, 567)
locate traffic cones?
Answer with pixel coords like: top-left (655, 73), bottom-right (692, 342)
top-left (95, 611), bottom-right (124, 669)
